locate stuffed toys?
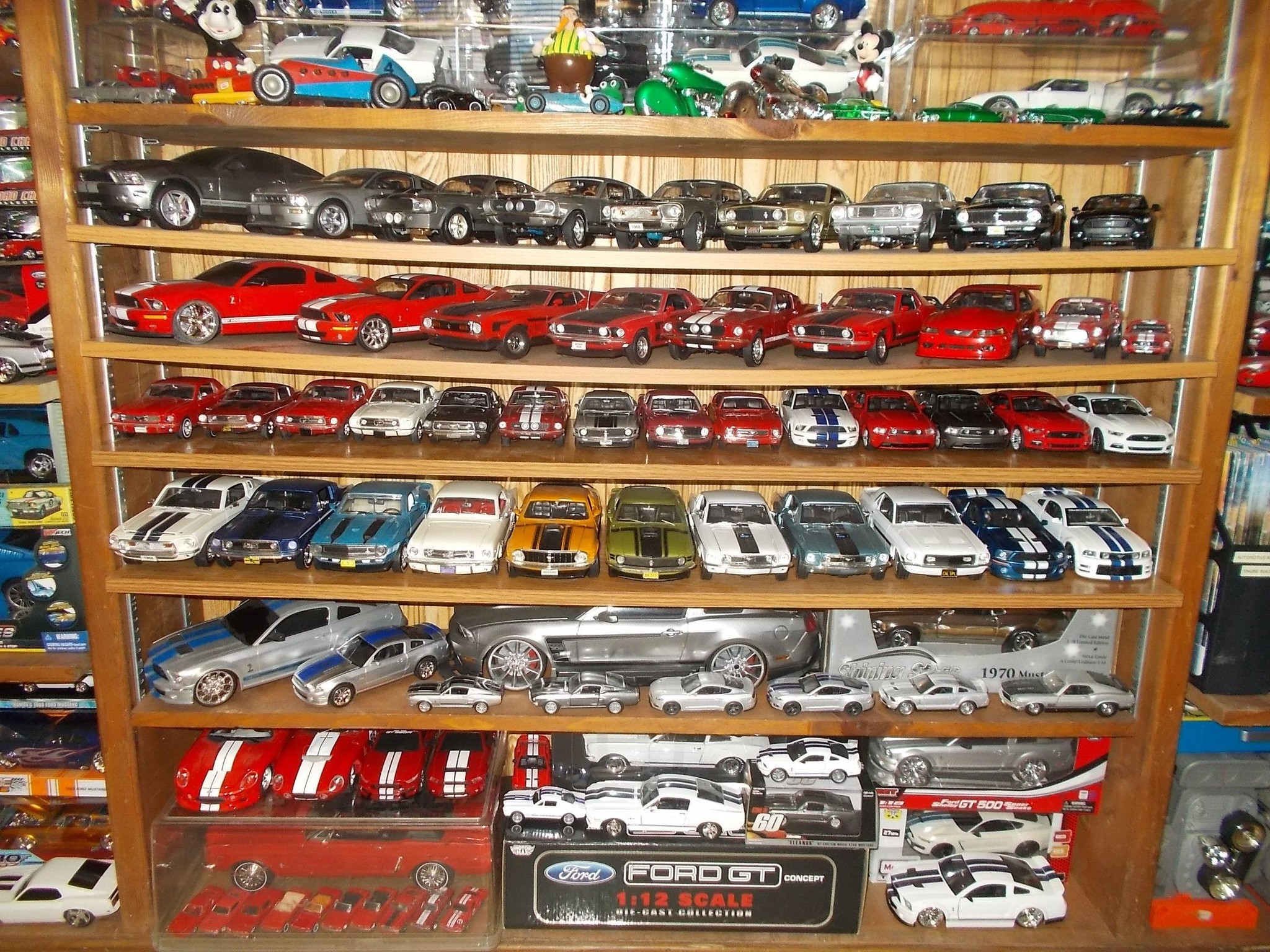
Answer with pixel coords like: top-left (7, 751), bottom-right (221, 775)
top-left (845, 21), bottom-right (896, 99)
top-left (172, 0), bottom-right (257, 77)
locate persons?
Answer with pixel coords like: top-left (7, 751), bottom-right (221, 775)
top-left (533, 5), bottom-right (607, 92)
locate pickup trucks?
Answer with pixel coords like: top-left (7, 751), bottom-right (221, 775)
top-left (962, 78), bottom-right (1199, 116)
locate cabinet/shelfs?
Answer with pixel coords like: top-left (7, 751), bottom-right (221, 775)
top-left (0, 0), bottom-right (1270, 952)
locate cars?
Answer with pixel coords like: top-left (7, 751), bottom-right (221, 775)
top-left (105, 260), bottom-right (1172, 369)
top-left (689, 0), bottom-right (867, 31)
top-left (483, 35), bottom-right (648, 91)
top-left (167, 825), bottom-right (492, 938)
top-left (108, 473), bottom-right (1153, 581)
top-left (68, 80), bottom-right (172, 105)
top-left (0, 857), bottom-right (119, 927)
top-left (0, 796), bottom-right (112, 854)
top-left (112, 374), bottom-right (1174, 455)
top-left (0, 417), bottom-right (63, 519)
top-left (501, 733), bottom-right (1073, 929)
top-left (0, 229), bottom-right (56, 385)
top-left (138, 599), bottom-right (1135, 718)
top-left (268, 26), bottom-right (445, 97)
top-left (818, 97), bottom-right (1106, 127)
top-left (683, 38), bottom-right (860, 94)
top-left (169, 728), bottom-right (496, 814)
top-left (955, 11), bottom-right (1167, 38)
top-left (73, 147), bottom-right (1161, 251)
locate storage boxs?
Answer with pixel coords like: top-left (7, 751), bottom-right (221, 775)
top-left (149, 730), bottom-right (511, 952)
top-left (868, 736), bottom-right (1112, 874)
top-left (0, 746), bottom-right (107, 798)
top-left (503, 829), bottom-right (871, 937)
top-left (0, 482), bottom-right (74, 528)
